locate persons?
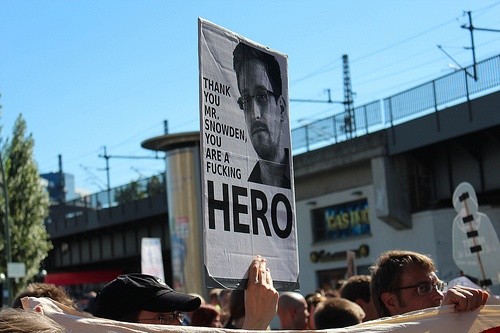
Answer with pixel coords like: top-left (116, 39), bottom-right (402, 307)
top-left (0, 252), bottom-right (500, 333)
top-left (94, 273), bottom-right (201, 326)
top-left (229, 42), bottom-right (292, 189)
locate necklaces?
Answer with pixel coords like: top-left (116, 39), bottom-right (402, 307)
top-left (372, 251), bottom-right (489, 333)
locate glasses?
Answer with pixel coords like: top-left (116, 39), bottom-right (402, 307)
top-left (396, 280), bottom-right (448, 296)
top-left (237, 89), bottom-right (275, 111)
top-left (136, 312), bottom-right (184, 325)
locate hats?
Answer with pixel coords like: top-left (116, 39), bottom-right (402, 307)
top-left (94, 273), bottom-right (201, 323)
